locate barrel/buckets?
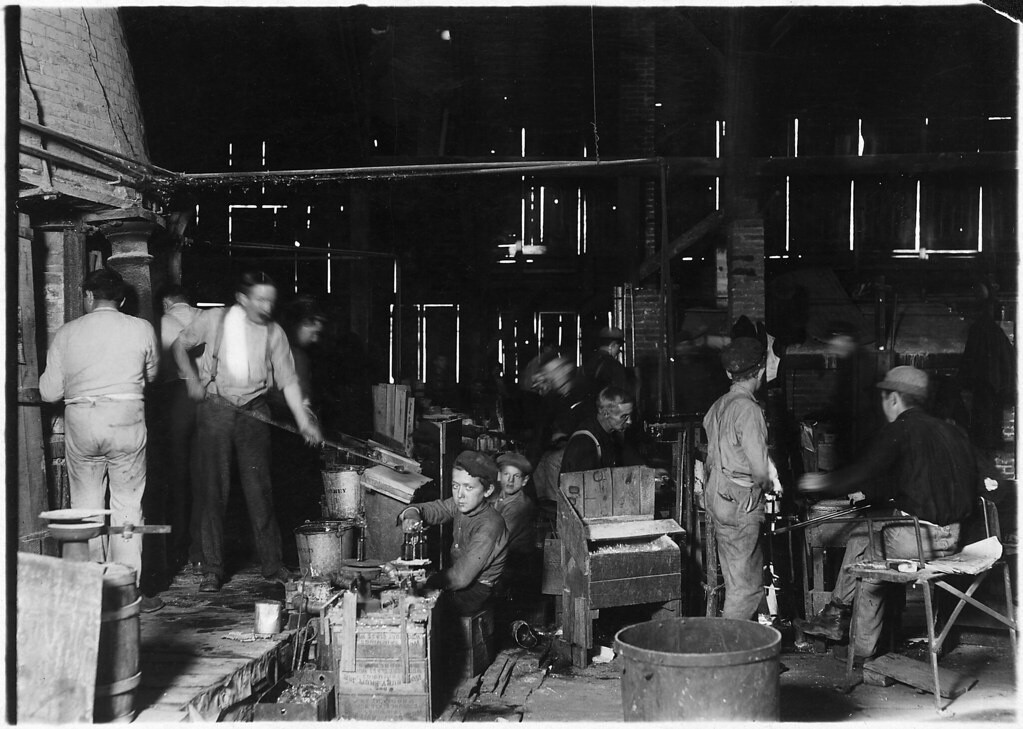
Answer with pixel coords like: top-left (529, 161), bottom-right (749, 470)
top-left (320, 464), bottom-right (366, 518)
top-left (615, 618), bottom-right (782, 722)
top-left (93, 563), bottom-right (142, 724)
top-left (254, 600), bottom-right (282, 634)
top-left (296, 521), bottom-right (357, 575)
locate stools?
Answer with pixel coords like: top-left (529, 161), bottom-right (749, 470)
top-left (831, 499), bottom-right (1017, 715)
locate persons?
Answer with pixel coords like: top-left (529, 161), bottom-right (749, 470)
top-left (468, 326), bottom-right (670, 518)
top-left (686, 329), bottom-right (785, 620)
top-left (489, 455), bottom-right (534, 594)
top-left (402, 451), bottom-right (509, 680)
top-left (798, 366), bottom-right (974, 669)
top-left (159, 271), bottom-right (324, 593)
top-left (39, 268), bottom-right (165, 613)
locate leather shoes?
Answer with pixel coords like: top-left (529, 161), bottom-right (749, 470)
top-left (265, 567), bottom-right (299, 581)
top-left (200, 572), bottom-right (220, 591)
top-left (834, 645), bottom-right (875, 667)
top-left (793, 601), bottom-right (846, 640)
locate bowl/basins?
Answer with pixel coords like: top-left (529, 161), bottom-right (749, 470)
top-left (655, 478), bottom-right (669, 493)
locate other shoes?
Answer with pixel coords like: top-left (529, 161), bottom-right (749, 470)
top-left (140, 593), bottom-right (165, 612)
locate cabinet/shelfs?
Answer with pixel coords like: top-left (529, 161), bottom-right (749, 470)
top-left (328, 589), bottom-right (442, 722)
top-left (541, 464), bottom-right (677, 672)
top-left (933, 547), bottom-right (1016, 655)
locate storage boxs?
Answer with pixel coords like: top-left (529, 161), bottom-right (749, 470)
top-left (254, 669), bottom-right (335, 722)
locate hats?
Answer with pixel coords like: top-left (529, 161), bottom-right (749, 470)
top-left (496, 453), bottom-right (532, 473)
top-left (721, 337), bottom-right (763, 373)
top-left (457, 451), bottom-right (497, 482)
top-left (876, 366), bottom-right (928, 398)
top-left (600, 327), bottom-right (624, 338)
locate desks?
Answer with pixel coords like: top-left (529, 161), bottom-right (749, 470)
top-left (805, 501), bottom-right (894, 646)
